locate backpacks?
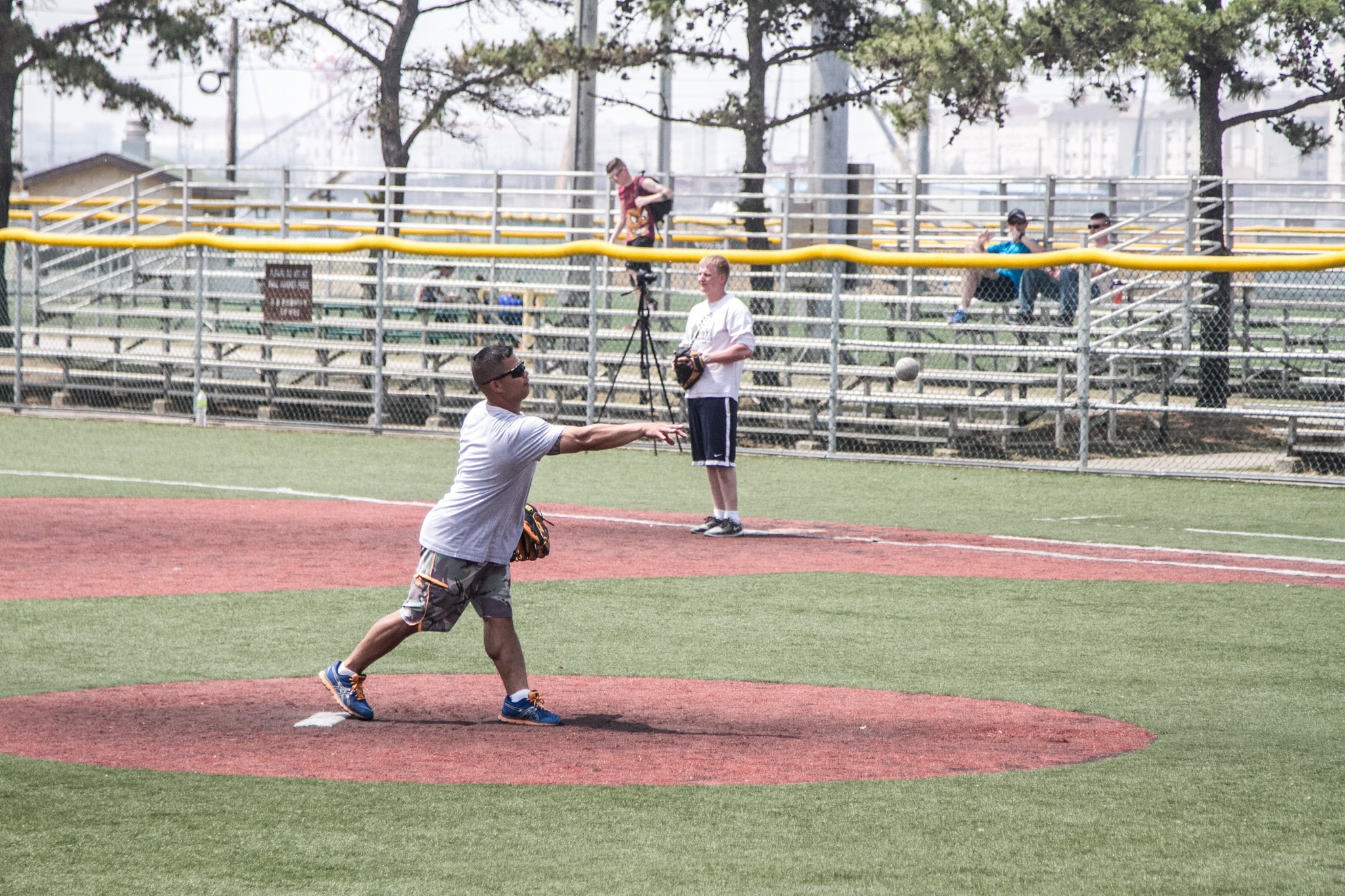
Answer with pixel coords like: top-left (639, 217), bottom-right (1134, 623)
top-left (637, 170), bottom-right (672, 221)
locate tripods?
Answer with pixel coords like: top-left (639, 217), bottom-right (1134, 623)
top-left (592, 290), bottom-right (685, 456)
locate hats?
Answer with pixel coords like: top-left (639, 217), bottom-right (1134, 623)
top-left (1008, 209), bottom-right (1025, 220)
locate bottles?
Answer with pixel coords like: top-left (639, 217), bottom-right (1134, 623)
top-left (195, 389), bottom-right (207, 427)
top-left (1112, 279), bottom-right (1123, 303)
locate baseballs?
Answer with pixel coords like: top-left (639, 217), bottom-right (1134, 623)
top-left (893, 357), bottom-right (918, 382)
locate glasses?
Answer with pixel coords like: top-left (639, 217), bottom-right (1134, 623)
top-left (482, 361), bottom-right (526, 385)
top-left (610, 169), bottom-right (624, 181)
top-left (1088, 224), bottom-right (1104, 229)
top-left (1008, 220), bottom-right (1027, 225)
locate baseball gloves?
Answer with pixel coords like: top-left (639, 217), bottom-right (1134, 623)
top-left (511, 503), bottom-right (551, 563)
top-left (672, 346), bottom-right (704, 392)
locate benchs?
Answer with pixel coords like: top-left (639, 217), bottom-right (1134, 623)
top-left (34, 163), bottom-right (1345, 475)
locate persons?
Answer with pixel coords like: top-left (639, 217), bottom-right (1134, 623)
top-left (414, 266), bottom-right (461, 344)
top-left (319, 344), bottom-right (691, 727)
top-left (605, 157), bottom-right (674, 330)
top-left (948, 207), bottom-right (1048, 323)
top-left (1005, 213), bottom-right (1120, 328)
top-left (673, 253), bottom-right (755, 537)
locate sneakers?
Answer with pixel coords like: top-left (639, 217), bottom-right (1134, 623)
top-left (690, 516), bottom-right (723, 534)
top-left (704, 518), bottom-right (743, 537)
top-left (498, 690), bottom-right (562, 726)
top-left (318, 661), bottom-right (374, 720)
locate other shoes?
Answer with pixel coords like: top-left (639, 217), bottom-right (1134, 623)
top-left (1013, 312), bottom-right (1034, 324)
top-left (623, 321), bottom-right (642, 329)
top-left (950, 310), bottom-right (970, 324)
top-left (649, 300), bottom-right (658, 318)
top-left (1057, 314), bottom-right (1075, 324)
